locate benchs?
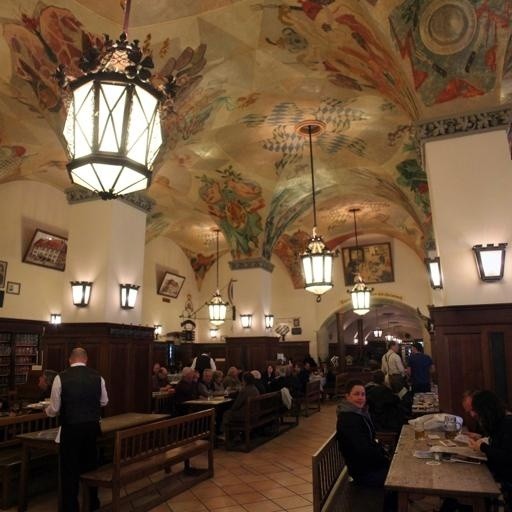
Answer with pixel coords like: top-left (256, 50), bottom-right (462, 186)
top-left (1, 366), bottom-right (100, 510)
top-left (312, 385), bottom-right (511, 511)
top-left (149, 366), bottom-right (384, 453)
top-left (81, 408), bottom-right (215, 511)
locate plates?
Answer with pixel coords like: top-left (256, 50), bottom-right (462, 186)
top-left (27, 400), bottom-right (50, 408)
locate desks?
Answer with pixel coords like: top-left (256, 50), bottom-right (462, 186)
top-left (17, 412), bottom-right (171, 509)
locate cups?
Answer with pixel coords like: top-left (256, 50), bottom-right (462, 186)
top-left (444, 415), bottom-right (462, 439)
top-left (414, 421), bottom-right (425, 440)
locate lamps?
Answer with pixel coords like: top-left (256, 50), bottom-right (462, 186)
top-left (472, 242), bottom-right (508, 281)
top-left (210, 329), bottom-right (218, 338)
top-left (204, 227), bottom-right (230, 327)
top-left (56, 1), bottom-right (171, 202)
top-left (239, 315), bottom-right (252, 328)
top-left (423, 257), bottom-right (443, 289)
top-left (68, 280), bottom-right (94, 308)
top-left (154, 325), bottom-right (162, 341)
top-left (345, 208), bottom-right (374, 317)
top-left (295, 120), bottom-right (335, 303)
top-left (265, 314), bottom-right (275, 329)
top-left (51, 314), bottom-right (62, 327)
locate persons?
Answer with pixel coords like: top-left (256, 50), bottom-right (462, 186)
top-left (42, 347), bottom-right (108, 512)
top-left (28, 369), bottom-right (58, 414)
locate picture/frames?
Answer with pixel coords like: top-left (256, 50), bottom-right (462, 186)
top-left (5, 281), bottom-right (22, 296)
top-left (341, 241), bottom-right (395, 286)
top-left (156, 271), bottom-right (186, 299)
top-left (21, 227), bottom-right (68, 272)
top-left (0, 260), bottom-right (7, 288)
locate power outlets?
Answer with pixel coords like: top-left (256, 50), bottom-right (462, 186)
top-left (119, 283), bottom-right (141, 310)
